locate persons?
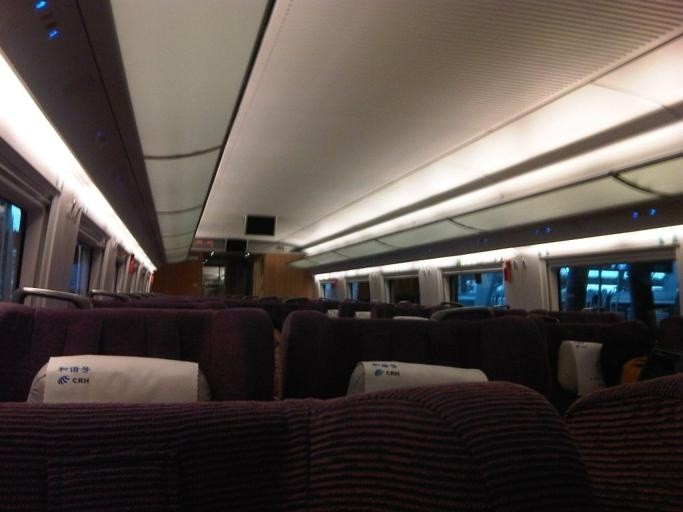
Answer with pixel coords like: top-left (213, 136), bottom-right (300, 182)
top-left (597, 320), bottom-right (654, 388)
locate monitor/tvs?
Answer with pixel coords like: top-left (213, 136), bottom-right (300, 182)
top-left (224, 237), bottom-right (249, 253)
top-left (244, 214), bottom-right (278, 237)
